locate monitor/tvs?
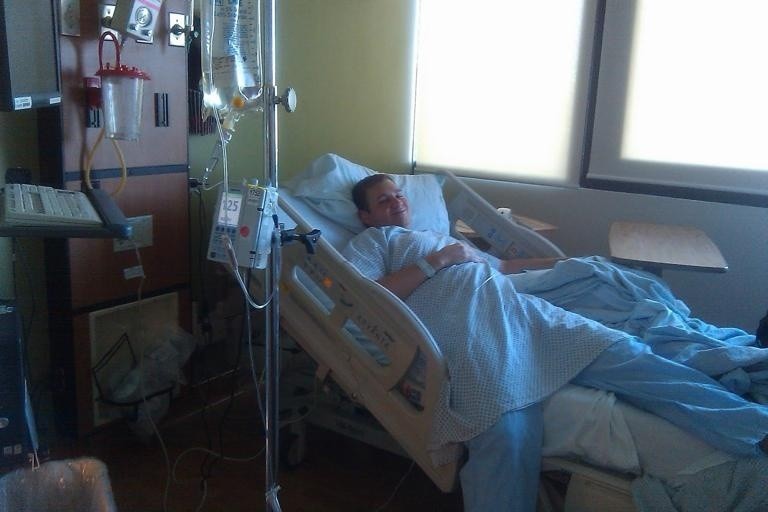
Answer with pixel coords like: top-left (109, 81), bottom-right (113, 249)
top-left (0, 0), bottom-right (64, 113)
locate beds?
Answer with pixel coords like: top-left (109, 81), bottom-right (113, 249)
top-left (220, 169), bottom-right (768, 512)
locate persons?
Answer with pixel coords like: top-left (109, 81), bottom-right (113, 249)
top-left (343, 174), bottom-right (764, 511)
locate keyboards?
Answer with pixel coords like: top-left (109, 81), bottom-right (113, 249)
top-left (4, 182), bottom-right (102, 226)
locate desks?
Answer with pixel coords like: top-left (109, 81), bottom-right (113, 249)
top-left (608, 220), bottom-right (731, 288)
